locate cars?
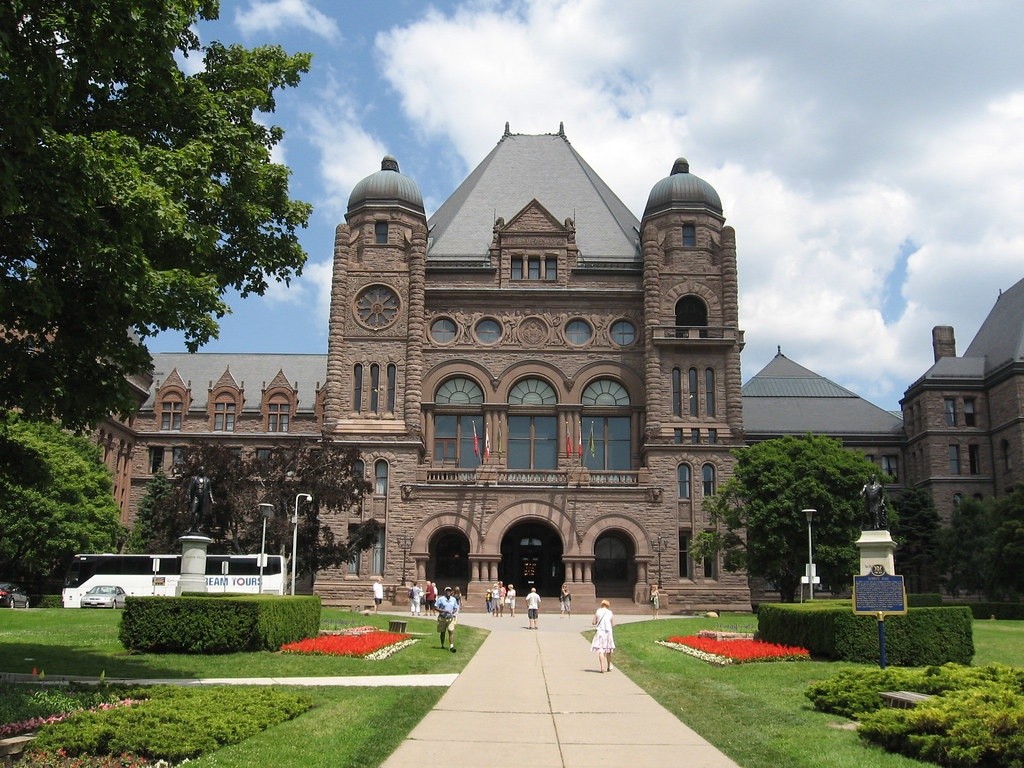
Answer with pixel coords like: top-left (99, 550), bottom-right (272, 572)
top-left (80, 584), bottom-right (129, 610)
top-left (0, 582), bottom-right (31, 609)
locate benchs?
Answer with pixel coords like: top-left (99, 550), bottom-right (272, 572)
top-left (387, 620), bottom-right (408, 633)
top-left (878, 691), bottom-right (931, 709)
top-left (0, 733), bottom-right (39, 768)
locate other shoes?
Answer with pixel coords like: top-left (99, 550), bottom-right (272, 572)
top-left (450, 647), bottom-right (456, 652)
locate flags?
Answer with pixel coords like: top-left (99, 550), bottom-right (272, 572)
top-left (498, 429), bottom-right (502, 459)
top-left (485, 428), bottom-right (490, 462)
top-left (579, 430), bottom-right (582, 457)
top-left (589, 432), bottom-right (594, 458)
top-left (474, 427), bottom-right (479, 456)
top-left (567, 428), bottom-right (572, 455)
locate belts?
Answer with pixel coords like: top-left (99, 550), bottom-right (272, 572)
top-left (439, 615), bottom-right (448, 618)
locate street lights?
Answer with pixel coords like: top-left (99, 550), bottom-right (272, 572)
top-left (650, 531), bottom-right (668, 590)
top-left (397, 529), bottom-right (414, 586)
top-left (801, 508), bottom-right (818, 600)
top-left (289, 493), bottom-right (312, 595)
top-left (258, 502), bottom-right (274, 595)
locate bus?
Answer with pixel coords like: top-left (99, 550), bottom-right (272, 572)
top-left (62, 553), bottom-right (287, 609)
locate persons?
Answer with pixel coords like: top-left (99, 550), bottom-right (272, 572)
top-left (451, 587), bottom-right (462, 621)
top-left (410, 582), bottom-right (423, 616)
top-left (485, 589), bottom-right (492, 613)
top-left (525, 588), bottom-right (541, 630)
top-left (491, 581), bottom-right (506, 617)
top-left (560, 584), bottom-right (570, 618)
top-left (592, 600), bottom-right (616, 673)
top-left (373, 579), bottom-right (383, 612)
top-left (858, 474), bottom-right (886, 530)
top-left (434, 587), bottom-right (457, 653)
top-left (185, 466), bottom-right (217, 533)
top-left (507, 584), bottom-right (516, 617)
top-left (423, 581), bottom-right (437, 616)
top-left (651, 586), bottom-right (660, 619)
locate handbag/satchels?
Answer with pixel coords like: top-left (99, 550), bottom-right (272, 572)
top-left (505, 598), bottom-right (510, 603)
top-left (595, 628), bottom-right (597, 633)
top-left (407, 589), bottom-right (414, 598)
top-left (420, 595), bottom-right (425, 604)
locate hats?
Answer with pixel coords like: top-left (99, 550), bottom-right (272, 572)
top-left (445, 586), bottom-right (451, 590)
